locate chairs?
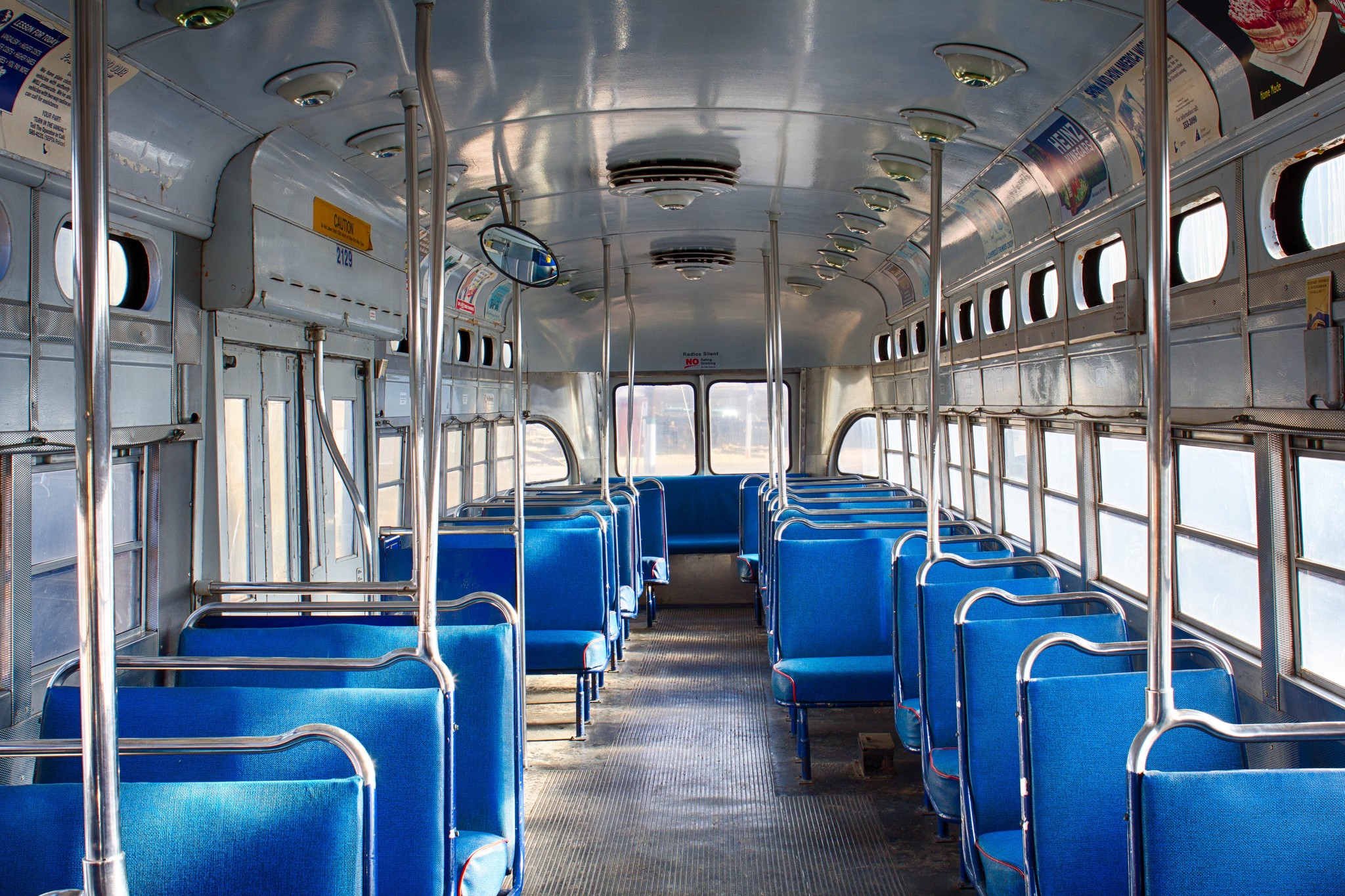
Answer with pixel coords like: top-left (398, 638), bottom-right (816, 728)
top-left (888, 529), bottom-right (1345, 896)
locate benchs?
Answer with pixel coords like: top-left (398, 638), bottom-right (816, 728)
top-left (595, 473), bottom-right (815, 556)
top-left (0, 584), bottom-right (532, 896)
top-left (756, 476), bottom-right (989, 781)
top-left (390, 478), bottom-right (674, 736)
